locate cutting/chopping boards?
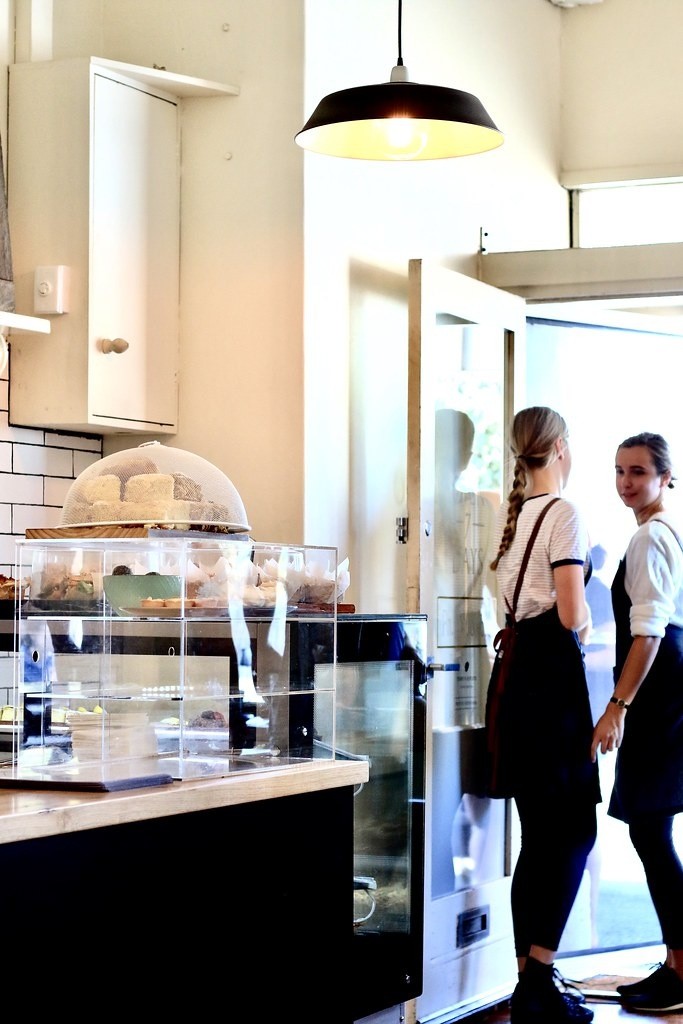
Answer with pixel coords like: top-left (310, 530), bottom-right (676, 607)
top-left (25, 528), bottom-right (249, 541)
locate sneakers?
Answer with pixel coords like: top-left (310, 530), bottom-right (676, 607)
top-left (510, 978), bottom-right (595, 1024)
top-left (618, 967), bottom-right (683, 1011)
top-left (517, 967), bottom-right (585, 1003)
top-left (618, 962), bottom-right (667, 996)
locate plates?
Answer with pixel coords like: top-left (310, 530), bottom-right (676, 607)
top-left (0, 598), bottom-right (28, 609)
top-left (29, 599), bottom-right (110, 610)
top-left (119, 607), bottom-right (228, 617)
top-left (243, 605), bottom-right (298, 617)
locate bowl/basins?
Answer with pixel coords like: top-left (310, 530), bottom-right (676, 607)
top-left (103, 575), bottom-right (185, 617)
top-left (181, 727), bottom-right (230, 753)
top-left (156, 726), bottom-right (180, 750)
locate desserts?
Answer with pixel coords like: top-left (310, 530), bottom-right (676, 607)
top-left (141, 596), bottom-right (218, 608)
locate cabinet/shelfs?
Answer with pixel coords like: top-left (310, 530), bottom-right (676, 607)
top-left (9, 61), bottom-right (180, 435)
top-left (0, 536), bottom-right (425, 1024)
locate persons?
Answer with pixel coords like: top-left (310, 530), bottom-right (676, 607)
top-left (479, 407), bottom-right (602, 1024)
top-left (591, 433), bottom-right (683, 1016)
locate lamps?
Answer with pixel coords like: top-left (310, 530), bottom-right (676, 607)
top-left (294, 0), bottom-right (504, 161)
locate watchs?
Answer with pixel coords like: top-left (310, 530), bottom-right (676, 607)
top-left (610, 696), bottom-right (630, 710)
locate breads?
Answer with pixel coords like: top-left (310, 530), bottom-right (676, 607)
top-left (64, 456), bottom-right (230, 523)
